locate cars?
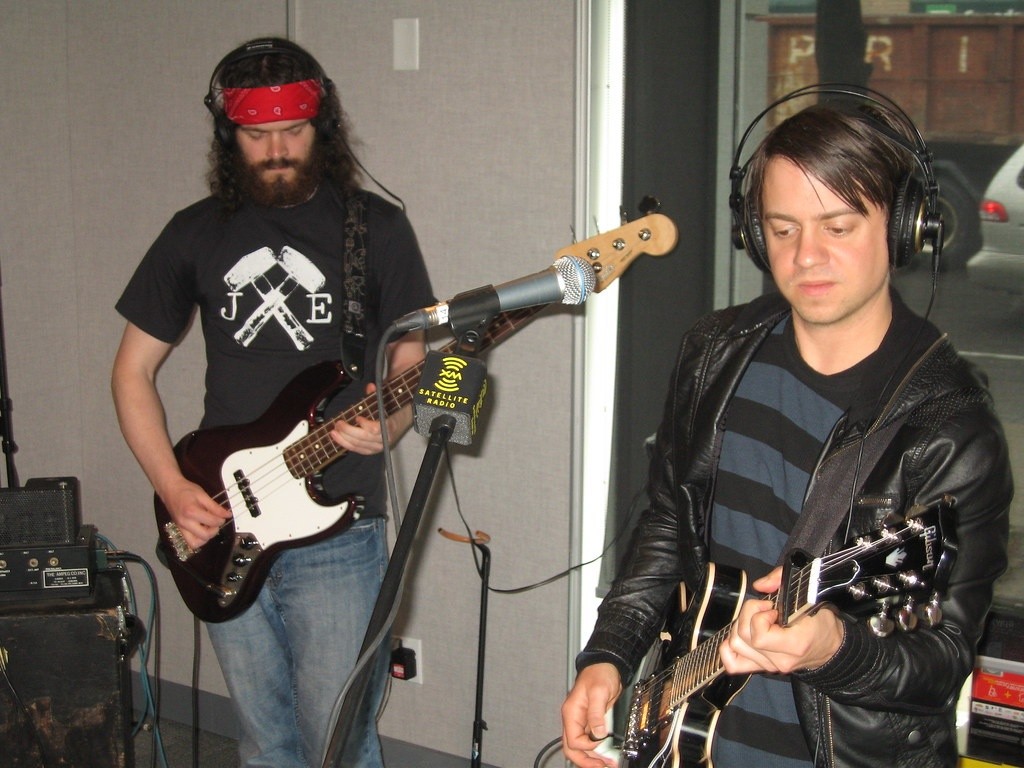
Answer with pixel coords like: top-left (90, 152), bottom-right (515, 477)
top-left (965, 144), bottom-right (1024, 296)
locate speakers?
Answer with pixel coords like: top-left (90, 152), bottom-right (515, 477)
top-left (0, 476), bottom-right (80, 547)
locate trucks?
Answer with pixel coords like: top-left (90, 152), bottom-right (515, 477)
top-left (767, 15), bottom-right (1024, 267)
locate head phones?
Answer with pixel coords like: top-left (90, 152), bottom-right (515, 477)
top-left (724, 85), bottom-right (944, 272)
top-left (202, 41), bottom-right (340, 150)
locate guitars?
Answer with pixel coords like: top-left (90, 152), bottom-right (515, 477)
top-left (156, 196), bottom-right (680, 626)
top-left (589, 493), bottom-right (961, 768)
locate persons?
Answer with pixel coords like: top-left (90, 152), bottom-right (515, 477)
top-left (561, 105), bottom-right (1014, 768)
top-left (113, 37), bottom-right (437, 768)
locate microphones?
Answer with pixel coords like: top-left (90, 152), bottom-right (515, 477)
top-left (390, 254), bottom-right (596, 333)
